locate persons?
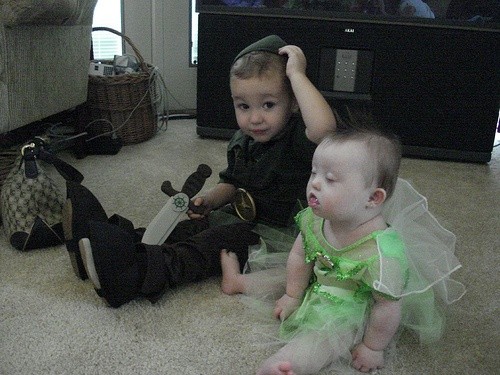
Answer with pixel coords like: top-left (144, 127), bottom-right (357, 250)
top-left (64, 35), bottom-right (345, 309)
top-left (221, 124), bottom-right (468, 375)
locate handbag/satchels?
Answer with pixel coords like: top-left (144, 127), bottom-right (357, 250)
top-left (2, 138), bottom-right (85, 251)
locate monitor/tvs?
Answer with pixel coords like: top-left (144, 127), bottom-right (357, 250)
top-left (196, 0), bottom-right (500, 34)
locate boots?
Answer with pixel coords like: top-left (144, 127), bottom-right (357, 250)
top-left (62, 198), bottom-right (126, 281)
top-left (78, 237), bottom-right (136, 308)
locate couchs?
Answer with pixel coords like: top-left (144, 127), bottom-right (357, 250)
top-left (0, 0), bottom-right (97, 133)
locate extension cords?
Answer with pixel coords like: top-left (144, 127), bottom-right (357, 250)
top-left (85, 136), bottom-right (123, 155)
